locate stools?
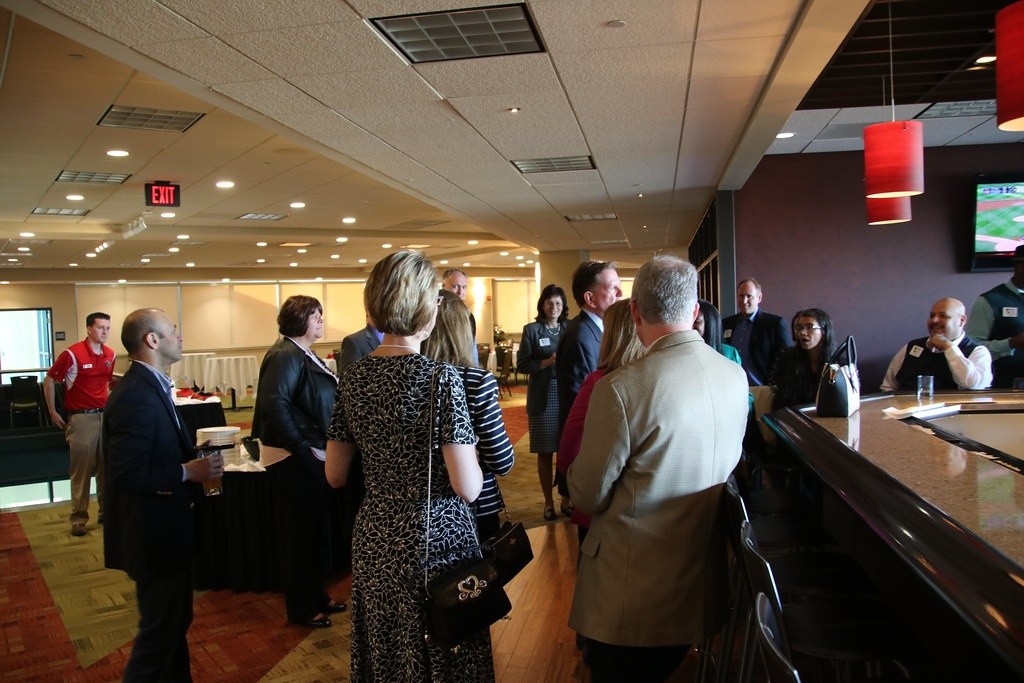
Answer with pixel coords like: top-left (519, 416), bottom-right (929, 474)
top-left (700, 440), bottom-right (876, 683)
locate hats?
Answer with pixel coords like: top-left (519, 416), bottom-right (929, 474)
top-left (1013, 245), bottom-right (1024, 260)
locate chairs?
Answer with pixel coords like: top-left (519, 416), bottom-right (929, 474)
top-left (478, 343), bottom-right (527, 398)
top-left (10, 375), bottom-right (64, 426)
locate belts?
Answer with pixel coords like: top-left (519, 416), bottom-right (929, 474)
top-left (72, 408), bottom-right (104, 414)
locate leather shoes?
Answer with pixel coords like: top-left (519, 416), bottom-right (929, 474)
top-left (320, 602), bottom-right (347, 614)
top-left (561, 507), bottom-right (573, 517)
top-left (305, 616), bottom-right (331, 628)
top-left (544, 509), bottom-right (555, 520)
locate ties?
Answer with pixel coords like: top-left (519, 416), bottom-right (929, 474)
top-left (157, 373), bottom-right (181, 430)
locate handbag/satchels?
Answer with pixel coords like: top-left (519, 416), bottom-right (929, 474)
top-left (416, 558), bottom-right (512, 652)
top-left (481, 521), bottom-right (534, 588)
top-left (816, 335), bottom-right (860, 418)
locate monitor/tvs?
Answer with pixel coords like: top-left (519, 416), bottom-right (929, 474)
top-left (966, 173), bottom-right (1024, 273)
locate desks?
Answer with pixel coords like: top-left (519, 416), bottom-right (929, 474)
top-left (0, 427), bottom-right (98, 507)
top-left (485, 350), bottom-right (499, 371)
top-left (171, 353), bottom-right (364, 594)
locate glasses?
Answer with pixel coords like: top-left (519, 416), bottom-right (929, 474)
top-left (795, 324), bottom-right (822, 331)
top-left (544, 301), bottom-right (563, 306)
top-left (435, 296), bottom-right (444, 305)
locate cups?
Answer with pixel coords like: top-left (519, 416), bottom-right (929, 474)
top-left (198, 450), bottom-right (222, 496)
top-left (917, 375), bottom-right (933, 398)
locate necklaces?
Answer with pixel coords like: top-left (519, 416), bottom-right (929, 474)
top-left (546, 323), bottom-right (561, 335)
top-left (378, 344), bottom-right (418, 352)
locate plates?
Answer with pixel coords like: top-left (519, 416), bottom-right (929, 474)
top-left (196, 426), bottom-right (242, 466)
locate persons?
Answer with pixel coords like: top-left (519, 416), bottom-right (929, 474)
top-left (517, 283), bottom-right (571, 521)
top-left (42, 312), bottom-right (114, 536)
top-left (882, 297), bottom-right (993, 393)
top-left (103, 308), bottom-right (224, 683)
top-left (422, 290), bottom-right (514, 547)
top-left (559, 300), bottom-right (648, 564)
top-left (983, 186), bottom-right (1010, 194)
top-left (252, 294), bottom-right (346, 627)
top-left (720, 277), bottom-right (790, 388)
top-left (963, 245), bottom-right (1024, 388)
top-left (323, 248), bottom-right (495, 683)
top-left (442, 269), bottom-right (479, 367)
top-left (337, 317), bottom-right (385, 378)
top-left (769, 307), bottom-right (849, 464)
top-left (692, 300), bottom-right (763, 470)
top-left (569, 256), bottom-right (749, 683)
top-left (555, 260), bottom-right (624, 497)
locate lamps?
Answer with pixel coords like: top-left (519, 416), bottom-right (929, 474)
top-left (996, 0), bottom-right (1024, 132)
top-left (864, 1), bottom-right (924, 226)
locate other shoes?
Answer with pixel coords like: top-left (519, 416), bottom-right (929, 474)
top-left (71, 525), bottom-right (87, 536)
top-left (98, 516), bottom-right (104, 524)
